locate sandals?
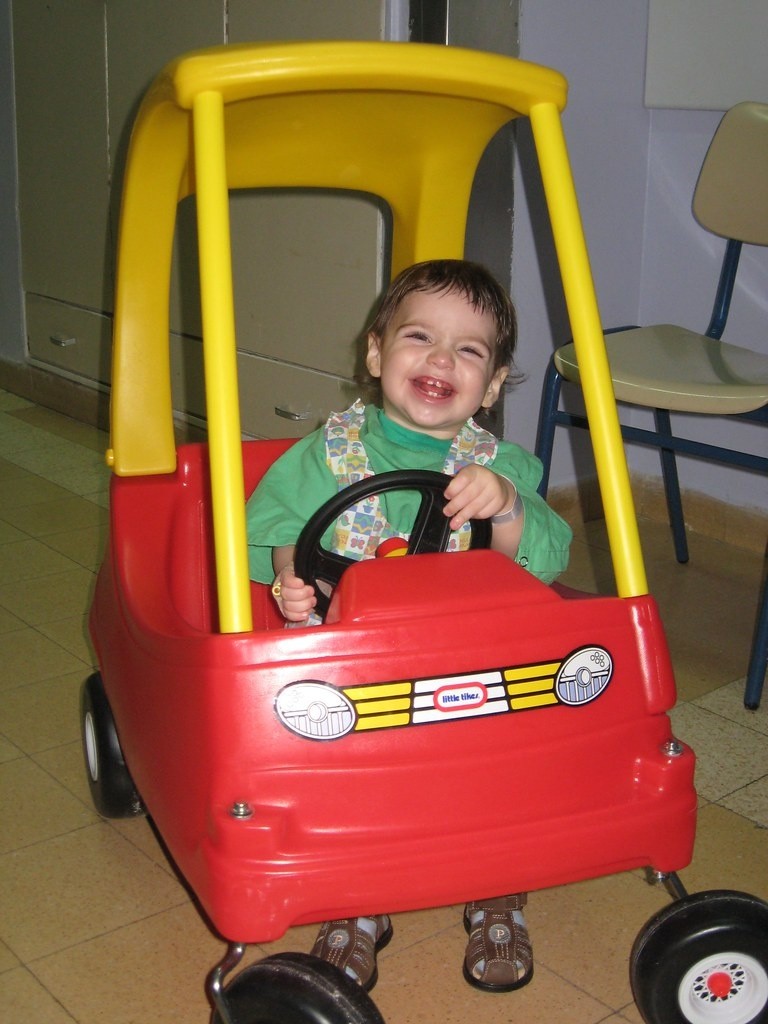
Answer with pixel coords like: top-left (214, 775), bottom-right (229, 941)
top-left (461, 890), bottom-right (535, 991)
top-left (309, 914), bottom-right (395, 991)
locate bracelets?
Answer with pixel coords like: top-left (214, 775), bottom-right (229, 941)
top-left (271, 564), bottom-right (293, 619)
top-left (490, 477), bottom-right (521, 524)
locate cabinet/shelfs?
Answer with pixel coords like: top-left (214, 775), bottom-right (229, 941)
top-left (11, 0), bottom-right (388, 441)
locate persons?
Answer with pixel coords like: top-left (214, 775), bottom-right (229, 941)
top-left (247, 261), bottom-right (573, 992)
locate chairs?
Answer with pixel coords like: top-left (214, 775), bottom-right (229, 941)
top-left (534, 100), bottom-right (768, 709)
